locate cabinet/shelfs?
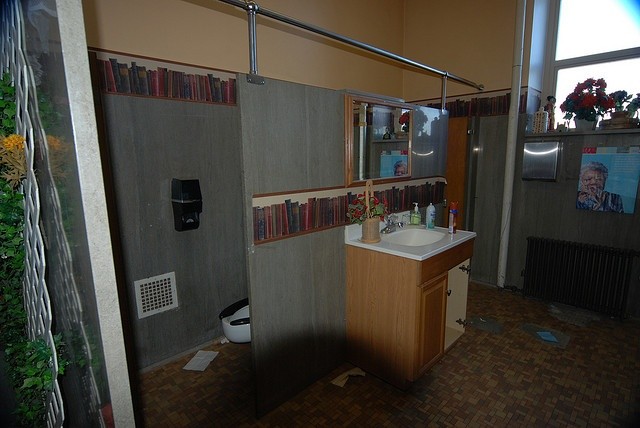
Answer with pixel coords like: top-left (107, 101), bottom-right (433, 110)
top-left (420, 238), bottom-right (474, 378)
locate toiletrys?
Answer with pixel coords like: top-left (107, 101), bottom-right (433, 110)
top-left (410, 202), bottom-right (423, 225)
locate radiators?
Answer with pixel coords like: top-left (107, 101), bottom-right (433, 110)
top-left (520, 237), bottom-right (637, 326)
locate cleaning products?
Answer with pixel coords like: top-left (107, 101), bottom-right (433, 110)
top-left (425, 203), bottom-right (436, 229)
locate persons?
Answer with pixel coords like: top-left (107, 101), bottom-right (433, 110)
top-left (544, 95), bottom-right (556, 131)
top-left (393, 161), bottom-right (409, 175)
top-left (577, 162), bottom-right (623, 211)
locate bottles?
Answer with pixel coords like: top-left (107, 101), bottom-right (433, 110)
top-left (533, 108), bottom-right (548, 134)
top-left (426, 203), bottom-right (435, 229)
top-left (411, 203), bottom-right (421, 226)
top-left (448, 201), bottom-right (458, 234)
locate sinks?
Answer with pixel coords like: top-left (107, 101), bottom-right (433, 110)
top-left (382, 229), bottom-right (445, 246)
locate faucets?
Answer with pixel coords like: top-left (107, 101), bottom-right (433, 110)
top-left (387, 219), bottom-right (404, 229)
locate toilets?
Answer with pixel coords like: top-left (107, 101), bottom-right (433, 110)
top-left (219, 298), bottom-right (251, 344)
